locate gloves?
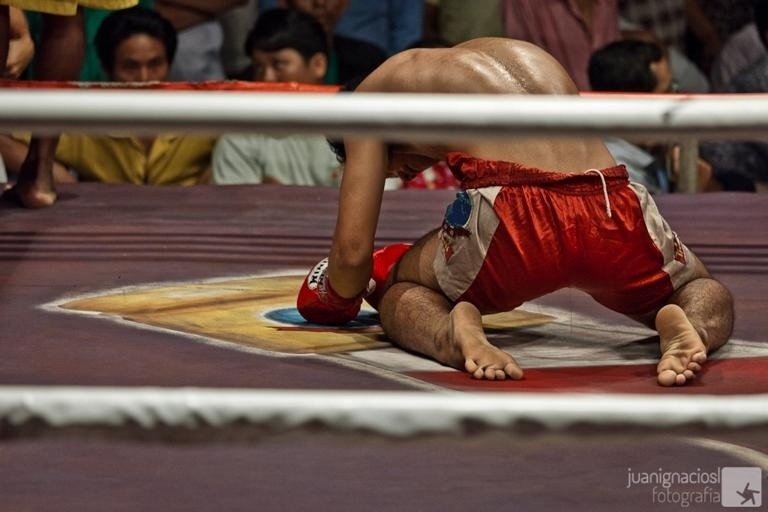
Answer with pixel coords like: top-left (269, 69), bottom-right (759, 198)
top-left (363, 243), bottom-right (413, 311)
top-left (297, 257), bottom-right (375, 324)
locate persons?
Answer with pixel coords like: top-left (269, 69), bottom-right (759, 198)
top-left (2, 0), bottom-right (767, 214)
top-left (296, 35), bottom-right (736, 389)
